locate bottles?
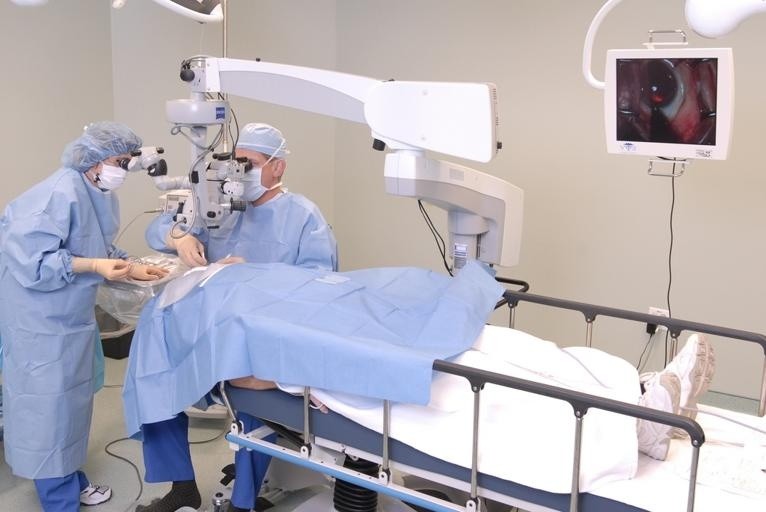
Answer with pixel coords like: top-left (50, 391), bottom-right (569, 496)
top-left (237, 123), bottom-right (290, 161)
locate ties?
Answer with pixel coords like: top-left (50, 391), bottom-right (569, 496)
top-left (604, 47), bottom-right (736, 161)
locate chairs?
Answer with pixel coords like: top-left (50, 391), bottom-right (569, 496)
top-left (80, 483), bottom-right (113, 507)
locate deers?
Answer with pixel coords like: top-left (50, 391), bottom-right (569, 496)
top-left (238, 157), bottom-right (287, 203)
top-left (89, 161), bottom-right (128, 192)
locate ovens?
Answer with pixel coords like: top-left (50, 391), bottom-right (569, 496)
top-left (192, 289), bottom-right (766, 512)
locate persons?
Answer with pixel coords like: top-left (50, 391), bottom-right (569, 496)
top-left (218, 327), bottom-right (716, 463)
top-left (2, 122), bottom-right (169, 512)
top-left (134, 122), bottom-right (336, 510)
